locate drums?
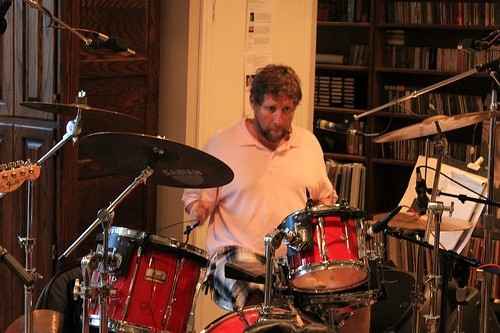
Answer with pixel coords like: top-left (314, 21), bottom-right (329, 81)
top-left (80, 226), bottom-right (210, 333)
top-left (278, 250), bottom-right (381, 300)
top-left (283, 204), bottom-right (371, 293)
top-left (200, 304), bottom-right (335, 333)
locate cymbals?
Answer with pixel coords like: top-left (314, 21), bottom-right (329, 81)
top-left (372, 111), bottom-right (500, 143)
top-left (19, 102), bottom-right (140, 124)
top-left (6, 309), bottom-right (65, 333)
top-left (78, 132), bottom-right (234, 189)
top-left (374, 212), bottom-right (474, 231)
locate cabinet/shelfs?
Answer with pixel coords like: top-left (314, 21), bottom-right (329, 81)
top-left (313, 0), bottom-right (500, 301)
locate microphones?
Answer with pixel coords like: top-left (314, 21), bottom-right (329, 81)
top-left (86, 39), bottom-right (110, 55)
top-left (316, 119), bottom-right (365, 136)
top-left (364, 206), bottom-right (402, 240)
top-left (457, 39), bottom-right (490, 49)
top-left (92, 31), bottom-right (135, 57)
top-left (184, 222), bottom-right (201, 234)
top-left (417, 168), bottom-right (430, 214)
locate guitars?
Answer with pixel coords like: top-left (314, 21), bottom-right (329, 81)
top-left (0, 158), bottom-right (40, 193)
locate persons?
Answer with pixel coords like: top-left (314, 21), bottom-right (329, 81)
top-left (181, 63), bottom-right (371, 333)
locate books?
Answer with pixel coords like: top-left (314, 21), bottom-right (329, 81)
top-left (314, 0), bottom-right (500, 298)
